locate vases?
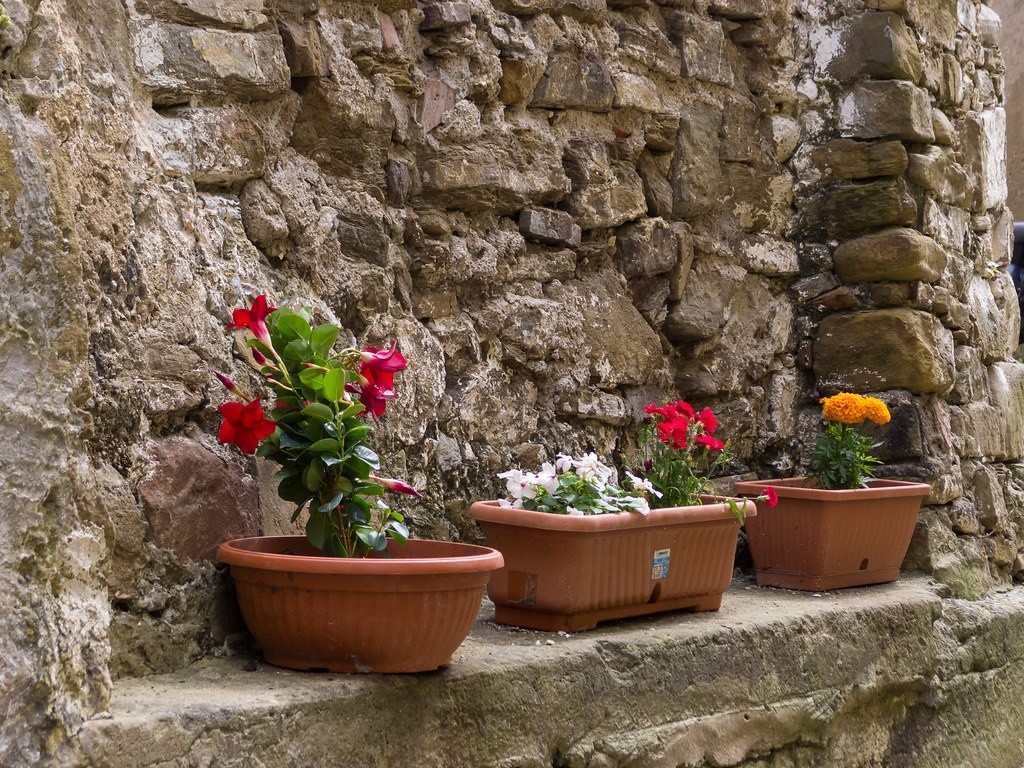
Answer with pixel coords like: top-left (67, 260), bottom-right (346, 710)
top-left (736, 474), bottom-right (931, 592)
top-left (218, 536), bottom-right (504, 676)
top-left (470, 498), bottom-right (756, 637)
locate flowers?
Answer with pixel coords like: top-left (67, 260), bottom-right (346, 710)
top-left (802, 391), bottom-right (891, 488)
top-left (215, 292), bottom-right (425, 559)
top-left (493, 400), bottom-right (779, 521)
